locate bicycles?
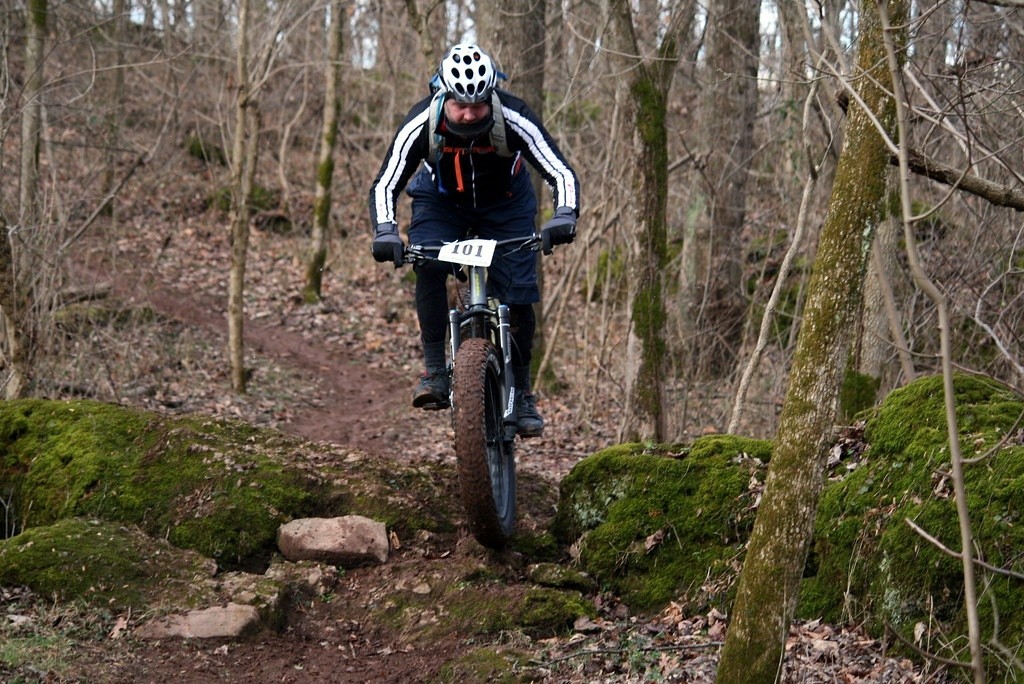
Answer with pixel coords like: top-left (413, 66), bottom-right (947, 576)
top-left (397, 233), bottom-right (569, 550)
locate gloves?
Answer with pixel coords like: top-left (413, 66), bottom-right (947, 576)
top-left (541, 206), bottom-right (576, 255)
top-left (369, 222), bottom-right (405, 269)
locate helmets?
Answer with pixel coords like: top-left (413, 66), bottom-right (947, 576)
top-left (437, 41), bottom-right (498, 104)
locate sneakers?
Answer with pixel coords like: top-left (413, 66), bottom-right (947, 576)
top-left (514, 391), bottom-right (545, 437)
top-left (407, 365), bottom-right (452, 411)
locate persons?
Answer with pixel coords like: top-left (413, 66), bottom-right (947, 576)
top-left (367, 42), bottom-right (582, 440)
